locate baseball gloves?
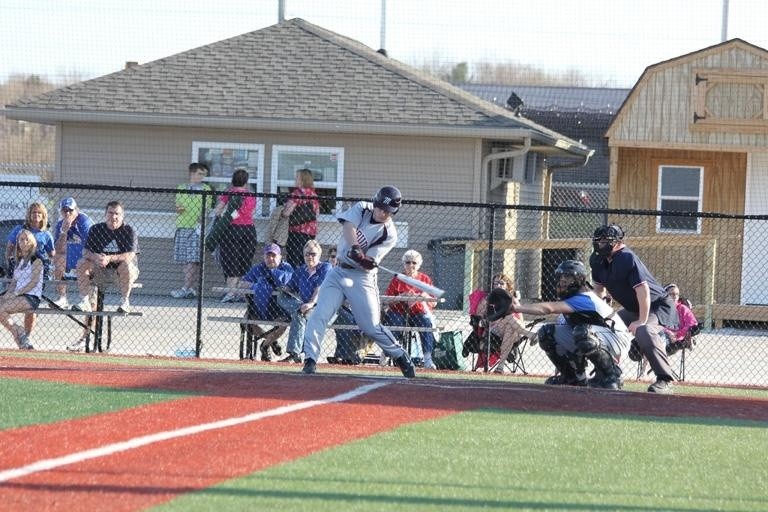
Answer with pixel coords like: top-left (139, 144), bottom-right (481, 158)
top-left (482, 288), bottom-right (515, 322)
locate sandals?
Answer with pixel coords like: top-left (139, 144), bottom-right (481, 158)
top-left (494, 366), bottom-right (504, 373)
top-left (529, 332), bottom-right (539, 346)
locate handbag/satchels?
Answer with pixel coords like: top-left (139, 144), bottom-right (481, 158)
top-left (289, 190), bottom-right (316, 226)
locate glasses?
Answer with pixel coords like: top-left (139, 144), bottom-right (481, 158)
top-left (63, 209), bottom-right (73, 212)
top-left (405, 261), bottom-right (417, 265)
top-left (304, 252), bottom-right (317, 257)
top-left (493, 280), bottom-right (507, 285)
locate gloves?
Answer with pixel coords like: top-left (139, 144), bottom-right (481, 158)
top-left (351, 244), bottom-right (364, 261)
top-left (361, 255), bottom-right (375, 270)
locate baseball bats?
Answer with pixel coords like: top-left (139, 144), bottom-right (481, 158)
top-left (345, 251), bottom-right (446, 298)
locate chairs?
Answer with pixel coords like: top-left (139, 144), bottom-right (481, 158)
top-left (637, 298), bottom-right (704, 382)
top-left (469, 290), bottom-right (546, 376)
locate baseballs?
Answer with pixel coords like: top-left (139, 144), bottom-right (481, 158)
top-left (339, 277), bottom-right (354, 288)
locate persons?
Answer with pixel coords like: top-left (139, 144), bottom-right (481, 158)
top-left (476, 274), bottom-right (539, 375)
top-left (509, 259), bottom-right (631, 391)
top-left (282, 169), bottom-right (321, 271)
top-left (237, 242), bottom-right (293, 362)
top-left (302, 184), bottom-right (416, 379)
top-left (169, 163), bottom-right (212, 299)
top-left (52, 196), bottom-right (97, 351)
top-left (326, 247), bottom-right (338, 268)
top-left (5, 201), bottom-right (55, 335)
top-left (589, 223), bottom-right (680, 394)
top-left (0, 228), bottom-right (43, 349)
top-left (379, 249), bottom-right (437, 369)
top-left (213, 169), bottom-right (256, 302)
top-left (276, 239), bottom-right (330, 364)
top-left (637, 283), bottom-right (699, 381)
top-left (71, 200), bottom-right (140, 315)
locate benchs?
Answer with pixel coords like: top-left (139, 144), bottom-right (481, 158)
top-left (0, 278), bottom-right (143, 353)
top-left (206, 316), bottom-right (445, 366)
top-left (20, 308), bottom-right (143, 353)
top-left (212, 286), bottom-right (446, 360)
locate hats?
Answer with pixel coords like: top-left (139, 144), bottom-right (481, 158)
top-left (58, 197), bottom-right (77, 211)
top-left (263, 243), bottom-right (281, 255)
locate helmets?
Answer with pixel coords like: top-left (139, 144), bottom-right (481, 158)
top-left (592, 224), bottom-right (625, 257)
top-left (372, 185), bottom-right (402, 213)
top-left (554, 260), bottom-right (587, 297)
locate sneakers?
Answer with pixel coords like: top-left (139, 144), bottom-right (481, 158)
top-left (277, 354), bottom-right (301, 364)
top-left (379, 352), bottom-right (393, 366)
top-left (116, 298), bottom-right (130, 312)
top-left (544, 370), bottom-right (588, 387)
top-left (14, 334), bottom-right (33, 349)
top-left (66, 338), bottom-right (92, 352)
top-left (48, 296), bottom-right (68, 309)
top-left (71, 298), bottom-right (92, 312)
top-left (636, 372), bottom-right (648, 380)
top-left (302, 358), bottom-right (317, 375)
top-left (424, 358), bottom-right (437, 370)
top-left (170, 288), bottom-right (196, 298)
top-left (303, 307), bottom-right (316, 321)
top-left (271, 341), bottom-right (282, 356)
top-left (647, 379), bottom-right (673, 394)
top-left (221, 294), bottom-right (240, 304)
top-left (590, 371), bottom-right (619, 391)
top-left (259, 340), bottom-right (272, 361)
top-left (396, 350), bottom-right (416, 378)
